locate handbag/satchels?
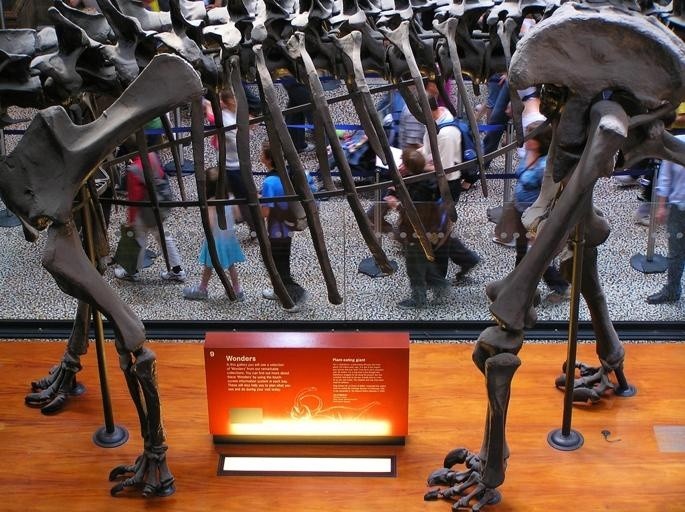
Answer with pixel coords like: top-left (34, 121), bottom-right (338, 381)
top-left (426, 192), bottom-right (450, 233)
top-left (277, 209), bottom-right (308, 232)
top-left (110, 227), bottom-right (140, 275)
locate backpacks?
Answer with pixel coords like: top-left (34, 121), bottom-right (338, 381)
top-left (438, 115), bottom-right (482, 178)
top-left (126, 151), bottom-right (172, 227)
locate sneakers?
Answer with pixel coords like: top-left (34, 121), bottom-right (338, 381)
top-left (114, 268), bottom-right (140, 282)
top-left (637, 193), bottom-right (649, 201)
top-left (182, 287), bottom-right (208, 299)
top-left (223, 288), bottom-right (243, 303)
top-left (616, 175), bottom-right (641, 185)
top-left (545, 292), bottom-right (568, 304)
top-left (298, 143), bottom-right (315, 152)
top-left (262, 289), bottom-right (281, 300)
top-left (636, 212), bottom-right (651, 227)
top-left (161, 268), bottom-right (187, 282)
top-left (397, 298), bottom-right (424, 309)
top-left (456, 255), bottom-right (480, 280)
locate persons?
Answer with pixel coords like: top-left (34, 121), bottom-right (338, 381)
top-left (380, 73), bottom-right (567, 307)
top-left (613, 100), bottom-right (685, 304)
top-left (105, 65), bottom-right (319, 304)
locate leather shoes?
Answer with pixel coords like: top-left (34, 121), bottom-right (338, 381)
top-left (647, 292), bottom-right (680, 303)
